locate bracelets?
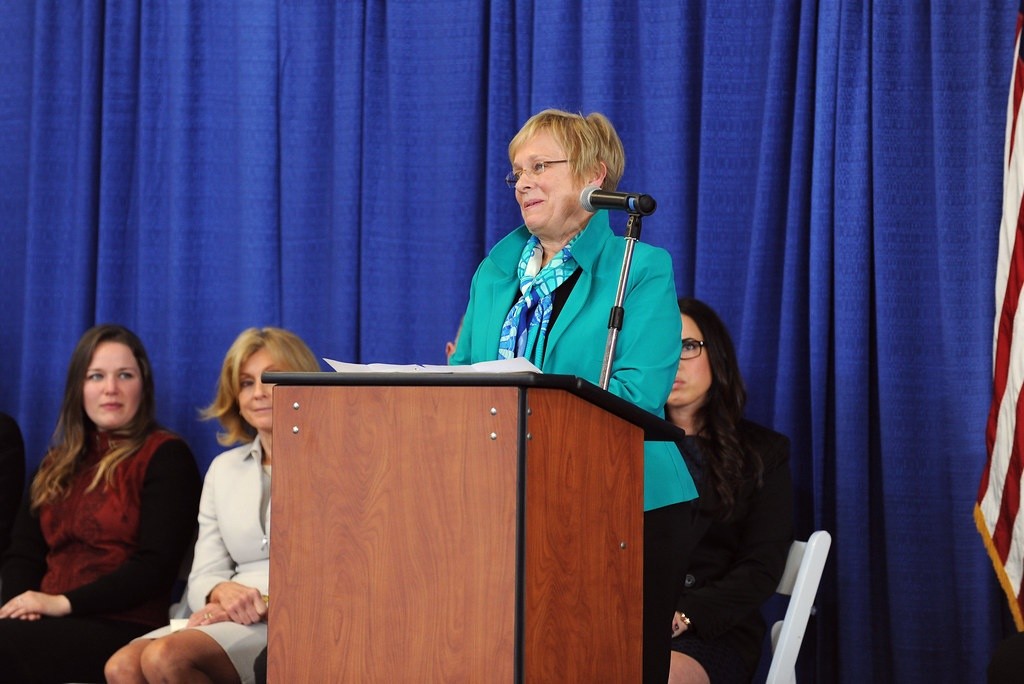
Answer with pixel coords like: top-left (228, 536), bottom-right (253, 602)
top-left (678, 611), bottom-right (691, 626)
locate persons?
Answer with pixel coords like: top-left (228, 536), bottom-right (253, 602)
top-left (104, 329), bottom-right (319, 684)
top-left (448, 109), bottom-right (699, 684)
top-left (664, 297), bottom-right (794, 684)
top-left (0, 325), bottom-right (205, 684)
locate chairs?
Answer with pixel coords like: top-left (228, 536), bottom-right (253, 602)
top-left (766, 530), bottom-right (832, 684)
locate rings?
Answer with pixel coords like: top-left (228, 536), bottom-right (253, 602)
top-left (204, 613), bottom-right (212, 619)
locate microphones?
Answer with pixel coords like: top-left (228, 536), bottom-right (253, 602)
top-left (580, 186), bottom-right (655, 213)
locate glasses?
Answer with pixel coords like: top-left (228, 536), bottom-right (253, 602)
top-left (505, 158), bottom-right (578, 188)
top-left (679, 337), bottom-right (708, 360)
